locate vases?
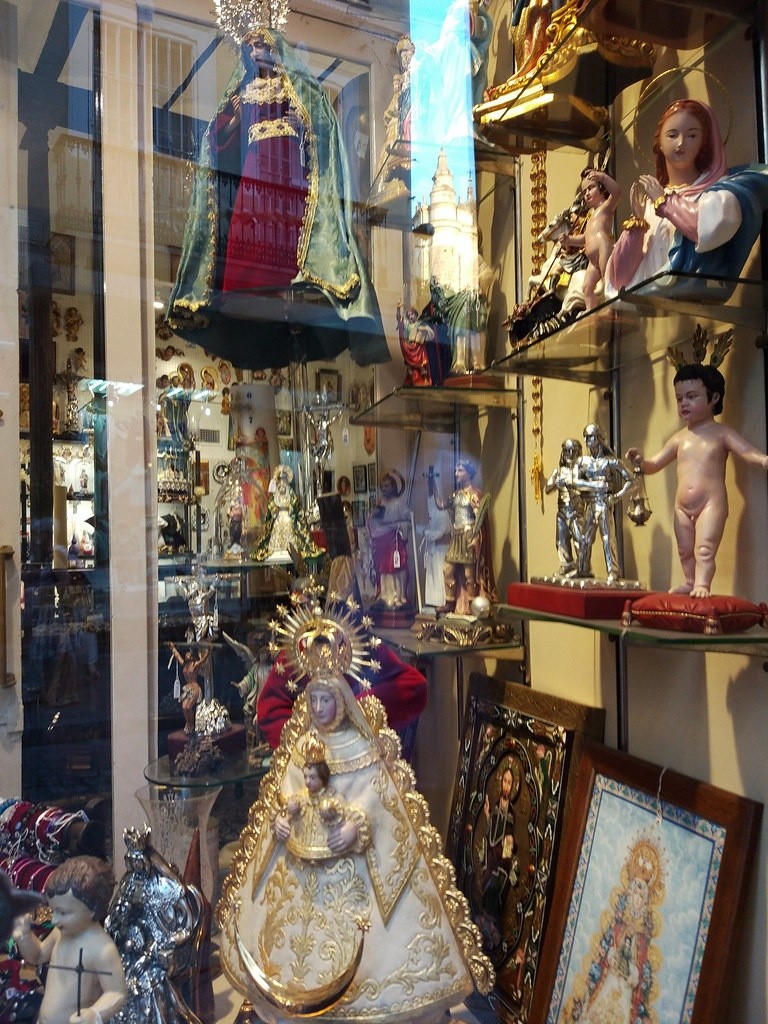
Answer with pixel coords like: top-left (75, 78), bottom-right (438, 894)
top-left (137, 774), bottom-right (223, 904)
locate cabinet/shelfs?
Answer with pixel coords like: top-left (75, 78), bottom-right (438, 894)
top-left (347, 383), bottom-right (527, 659)
top-left (492, 0), bottom-right (768, 742)
top-left (13, 372), bottom-right (96, 504)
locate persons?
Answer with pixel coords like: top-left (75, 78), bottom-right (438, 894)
top-left (396, 298), bottom-right (434, 387)
top-left (209, 0), bottom-right (338, 302)
top-left (423, 459), bottom-right (496, 617)
top-left (605, 98), bottom-right (768, 317)
top-left (396, 33), bottom-right (428, 155)
top-left (409, 490), bottom-right (454, 608)
top-left (545, 439), bottom-right (594, 578)
top-left (365, 469), bottom-right (413, 607)
top-left (213, 587), bottom-right (495, 1024)
top-left (175, 573), bottom-right (221, 668)
top-left (168, 641), bottom-right (211, 733)
top-left (227, 496), bottom-right (247, 553)
top-left (572, 423), bottom-right (639, 580)
top-left (376, 72), bottom-right (404, 175)
top-left (103, 824), bottom-right (193, 1024)
top-left (12, 855), bottom-right (127, 1024)
top-left (626, 364), bottom-right (768, 598)
top-left (222, 628), bottom-right (275, 748)
top-left (558, 170), bottom-right (623, 319)
top-left (251, 463), bottom-right (327, 564)
top-left (430, 218), bottom-right (494, 374)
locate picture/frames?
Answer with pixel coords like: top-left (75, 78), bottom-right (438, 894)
top-left (527, 736), bottom-right (765, 1024)
top-left (352, 465), bottom-right (367, 494)
top-left (17, 225), bottom-right (77, 295)
top-left (368, 463), bottom-right (377, 493)
top-left (314, 369), bottom-right (343, 405)
top-left (349, 502), bottom-right (365, 527)
top-left (444, 670), bottom-right (608, 1024)
